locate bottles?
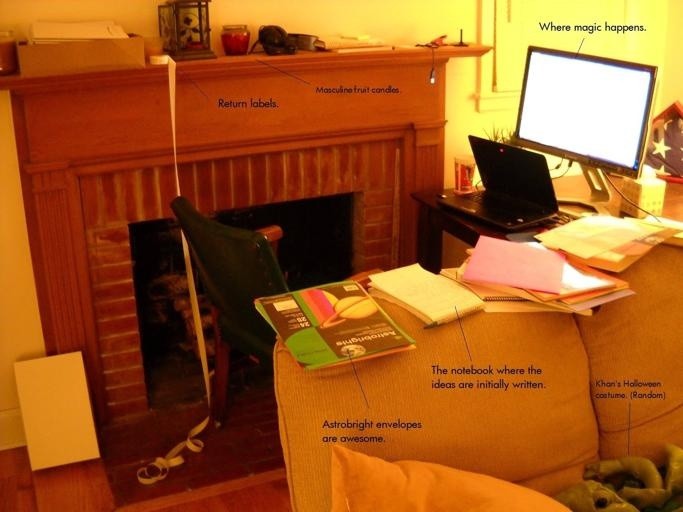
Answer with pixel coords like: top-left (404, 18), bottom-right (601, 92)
top-left (221, 24), bottom-right (251, 55)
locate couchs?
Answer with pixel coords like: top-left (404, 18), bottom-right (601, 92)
top-left (271, 243), bottom-right (682, 512)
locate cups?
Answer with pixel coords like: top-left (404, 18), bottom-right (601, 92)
top-left (452, 153), bottom-right (476, 196)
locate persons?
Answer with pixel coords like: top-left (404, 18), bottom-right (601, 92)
top-left (547, 442), bottom-right (683, 512)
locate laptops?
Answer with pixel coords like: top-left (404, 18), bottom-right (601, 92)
top-left (438, 133), bottom-right (558, 236)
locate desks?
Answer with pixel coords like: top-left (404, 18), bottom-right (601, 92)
top-left (408, 173), bottom-right (682, 275)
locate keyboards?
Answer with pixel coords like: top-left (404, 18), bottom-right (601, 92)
top-left (529, 211), bottom-right (574, 237)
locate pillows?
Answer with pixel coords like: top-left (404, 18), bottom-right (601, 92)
top-left (328, 443), bottom-right (571, 512)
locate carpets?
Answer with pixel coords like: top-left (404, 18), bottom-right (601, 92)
top-left (120, 465), bottom-right (292, 512)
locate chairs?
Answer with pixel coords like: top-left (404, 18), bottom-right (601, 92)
top-left (169, 196), bottom-right (384, 429)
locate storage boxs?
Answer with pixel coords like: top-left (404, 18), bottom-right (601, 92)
top-left (13, 29), bottom-right (150, 82)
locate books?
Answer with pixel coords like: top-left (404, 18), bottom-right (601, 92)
top-left (314, 34), bottom-right (397, 54)
top-left (244, 211), bottom-right (683, 372)
top-left (26, 20), bottom-right (129, 44)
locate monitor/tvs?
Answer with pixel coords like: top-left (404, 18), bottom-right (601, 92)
top-left (511, 43), bottom-right (661, 215)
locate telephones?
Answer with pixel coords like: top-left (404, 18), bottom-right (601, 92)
top-left (258, 24), bottom-right (294, 56)
